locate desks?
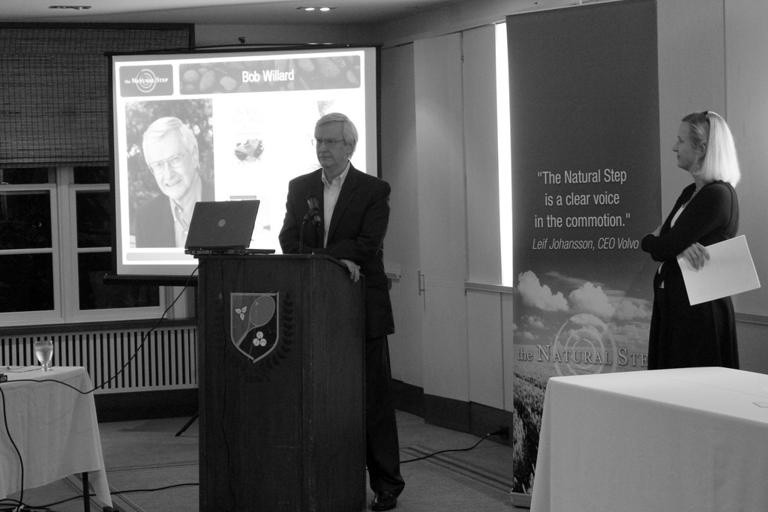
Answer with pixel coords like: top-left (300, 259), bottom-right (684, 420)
top-left (1, 365), bottom-right (111, 512)
top-left (526, 366), bottom-right (767, 510)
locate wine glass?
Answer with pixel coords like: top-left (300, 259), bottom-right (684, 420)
top-left (34, 340), bottom-right (54, 373)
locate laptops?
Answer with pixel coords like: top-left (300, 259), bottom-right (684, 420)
top-left (184, 200), bottom-right (275, 256)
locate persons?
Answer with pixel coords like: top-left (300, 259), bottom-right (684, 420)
top-left (278, 112), bottom-right (407, 512)
top-left (640, 109), bottom-right (741, 370)
top-left (135, 115), bottom-right (215, 248)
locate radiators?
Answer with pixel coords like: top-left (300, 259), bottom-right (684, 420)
top-left (0, 326), bottom-right (200, 395)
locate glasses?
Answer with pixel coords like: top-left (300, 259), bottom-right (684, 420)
top-left (312, 138), bottom-right (344, 147)
top-left (150, 150), bottom-right (189, 175)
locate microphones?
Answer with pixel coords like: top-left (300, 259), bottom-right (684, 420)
top-left (305, 190), bottom-right (322, 227)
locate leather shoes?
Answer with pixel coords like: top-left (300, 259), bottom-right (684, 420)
top-left (370, 493), bottom-right (396, 510)
